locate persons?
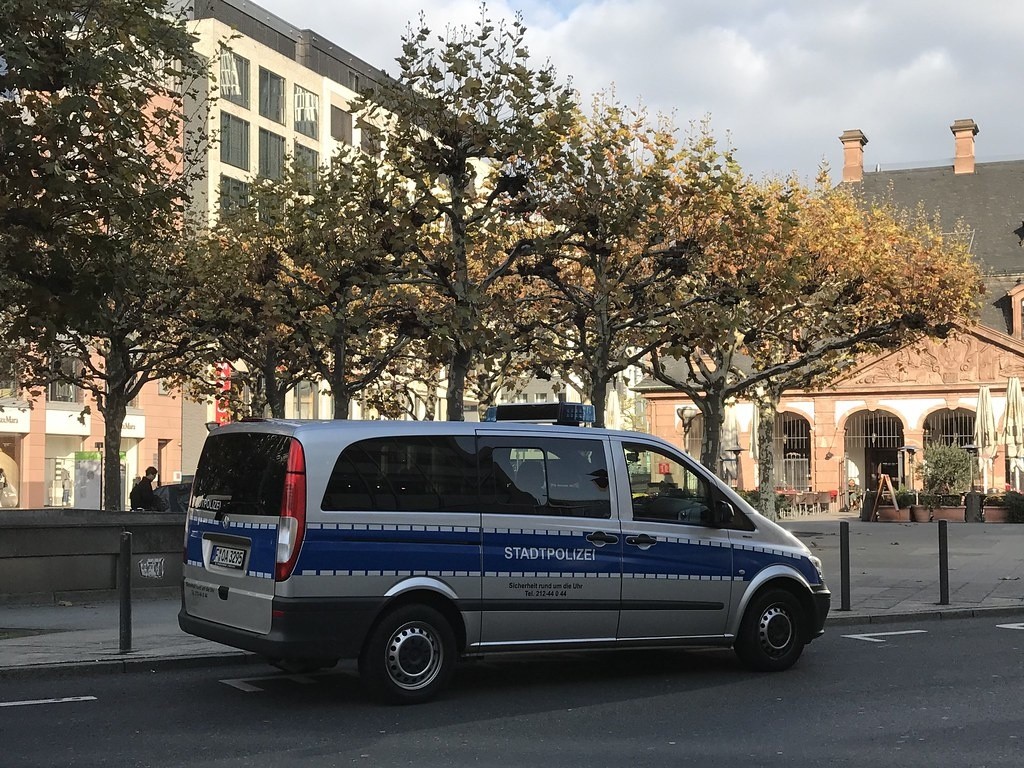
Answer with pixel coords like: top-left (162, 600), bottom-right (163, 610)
top-left (62, 473), bottom-right (70, 506)
top-left (87, 470), bottom-right (94, 484)
top-left (848, 479), bottom-right (861, 510)
top-left (131, 467), bottom-right (161, 512)
top-left (0, 468), bottom-right (18, 508)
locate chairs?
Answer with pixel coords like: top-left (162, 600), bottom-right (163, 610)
top-left (516, 460), bottom-right (547, 513)
top-left (543, 461), bottom-right (587, 516)
top-left (779, 491), bottom-right (830, 519)
top-left (332, 469), bottom-right (505, 512)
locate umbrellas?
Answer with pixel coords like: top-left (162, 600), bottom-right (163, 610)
top-left (749, 387), bottom-right (762, 491)
top-left (1001, 377), bottom-right (1024, 494)
top-left (719, 396), bottom-right (738, 487)
top-left (974, 386), bottom-right (1001, 495)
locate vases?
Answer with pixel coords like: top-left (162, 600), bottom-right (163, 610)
top-left (910, 505), bottom-right (931, 523)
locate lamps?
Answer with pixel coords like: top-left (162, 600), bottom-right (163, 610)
top-left (827, 452), bottom-right (834, 460)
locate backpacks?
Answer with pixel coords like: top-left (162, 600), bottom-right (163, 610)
top-left (130, 482), bottom-right (147, 510)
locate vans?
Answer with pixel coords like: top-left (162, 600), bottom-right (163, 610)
top-left (180, 402), bottom-right (833, 707)
